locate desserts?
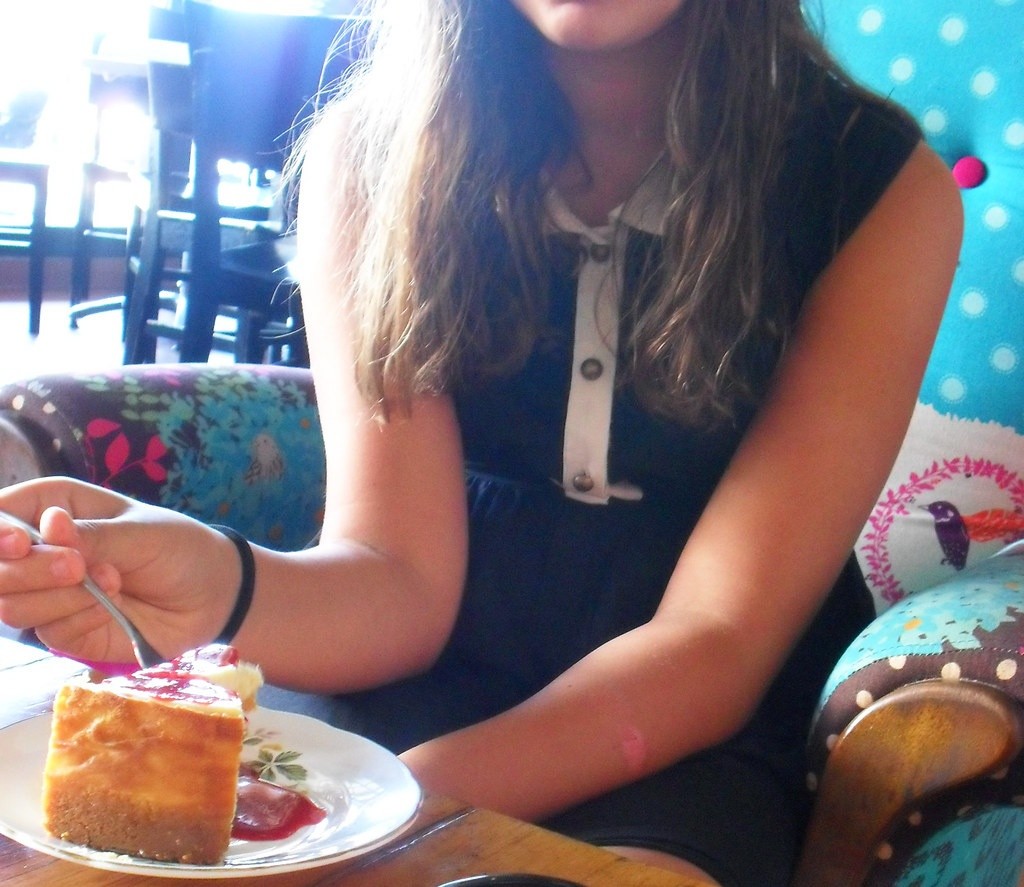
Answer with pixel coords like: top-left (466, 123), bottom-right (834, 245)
top-left (44, 643), bottom-right (264, 866)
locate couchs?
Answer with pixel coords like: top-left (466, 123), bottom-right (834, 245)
top-left (0, 1), bottom-right (1024, 886)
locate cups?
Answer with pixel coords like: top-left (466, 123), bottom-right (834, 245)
top-left (438, 871), bottom-right (585, 886)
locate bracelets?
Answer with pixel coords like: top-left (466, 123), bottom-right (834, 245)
top-left (207, 522), bottom-right (256, 648)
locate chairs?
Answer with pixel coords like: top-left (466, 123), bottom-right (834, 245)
top-left (0, 1), bottom-right (378, 364)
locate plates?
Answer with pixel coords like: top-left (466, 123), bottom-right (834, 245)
top-left (0, 707), bottom-right (425, 878)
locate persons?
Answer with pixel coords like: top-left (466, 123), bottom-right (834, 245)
top-left (0, 0), bottom-right (963, 886)
